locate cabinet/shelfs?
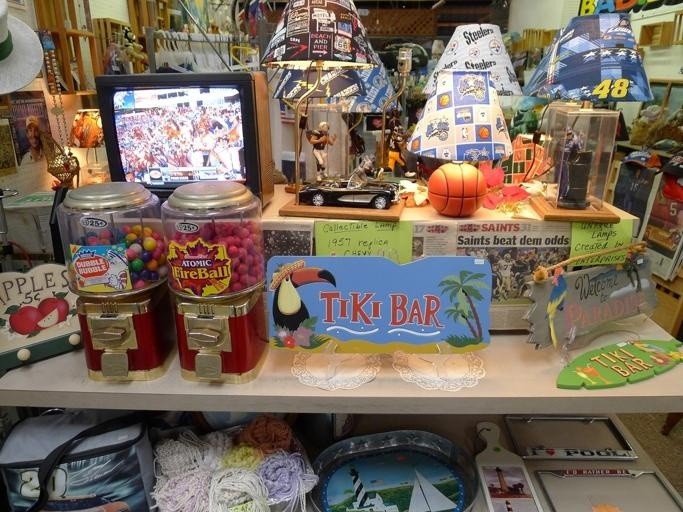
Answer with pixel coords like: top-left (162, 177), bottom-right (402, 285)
top-left (1, 183), bottom-right (683, 512)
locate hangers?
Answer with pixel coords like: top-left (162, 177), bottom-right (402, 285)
top-left (141, 29), bottom-right (259, 74)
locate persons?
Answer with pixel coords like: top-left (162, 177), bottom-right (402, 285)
top-left (310, 122), bottom-right (336, 180)
top-left (554, 125), bottom-right (585, 200)
top-left (497, 254), bottom-right (517, 297)
top-left (21, 116), bottom-right (48, 163)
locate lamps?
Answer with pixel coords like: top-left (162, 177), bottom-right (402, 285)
top-left (68, 109), bottom-right (105, 183)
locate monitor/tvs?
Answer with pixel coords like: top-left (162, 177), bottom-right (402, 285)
top-left (95, 69), bottom-right (273, 207)
